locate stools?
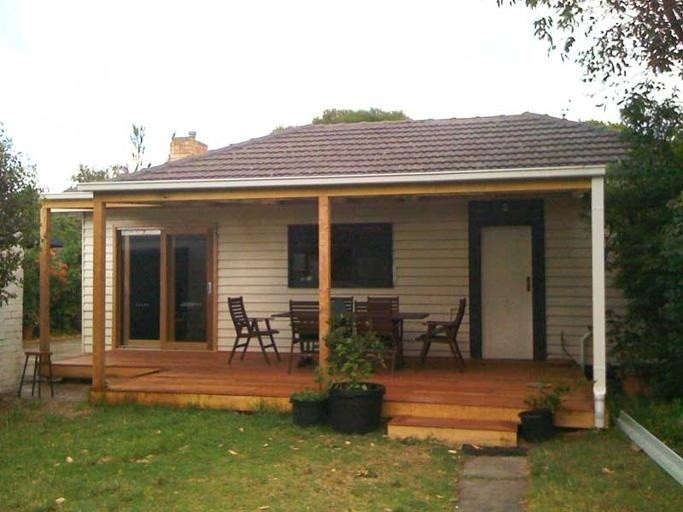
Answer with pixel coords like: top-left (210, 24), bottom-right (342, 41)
top-left (14, 349), bottom-right (58, 401)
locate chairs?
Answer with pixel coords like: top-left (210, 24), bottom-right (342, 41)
top-left (324, 295), bottom-right (356, 335)
top-left (364, 296), bottom-right (405, 352)
top-left (286, 297), bottom-right (320, 374)
top-left (412, 296), bottom-right (468, 372)
top-left (223, 294), bottom-right (281, 366)
top-left (353, 298), bottom-right (399, 375)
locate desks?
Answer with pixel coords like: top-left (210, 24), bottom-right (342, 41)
top-left (268, 311), bottom-right (430, 370)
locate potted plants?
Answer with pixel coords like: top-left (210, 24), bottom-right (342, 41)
top-left (358, 333), bottom-right (392, 429)
top-left (514, 372), bottom-right (599, 445)
top-left (321, 310), bottom-right (381, 436)
top-left (286, 386), bottom-right (329, 428)
